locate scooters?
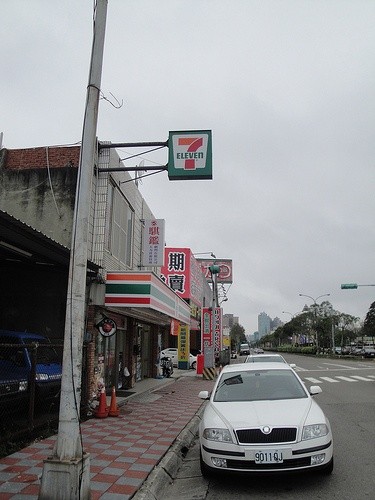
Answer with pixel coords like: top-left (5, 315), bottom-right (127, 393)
top-left (160, 352), bottom-right (175, 378)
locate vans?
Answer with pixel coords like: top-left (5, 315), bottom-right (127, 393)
top-left (0, 331), bottom-right (61, 404)
top-left (239, 344), bottom-right (250, 356)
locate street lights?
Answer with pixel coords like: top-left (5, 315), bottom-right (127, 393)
top-left (218, 298), bottom-right (228, 304)
top-left (282, 310), bottom-right (304, 319)
top-left (299, 293), bottom-right (331, 355)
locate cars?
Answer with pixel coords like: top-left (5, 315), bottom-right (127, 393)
top-left (197, 362), bottom-right (334, 480)
top-left (254, 348), bottom-right (264, 354)
top-left (244, 354), bottom-right (296, 370)
top-left (321, 345), bottom-right (375, 359)
top-left (160, 348), bottom-right (197, 369)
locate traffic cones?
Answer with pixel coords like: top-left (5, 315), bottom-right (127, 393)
top-left (94, 387), bottom-right (108, 418)
top-left (108, 385), bottom-right (120, 417)
